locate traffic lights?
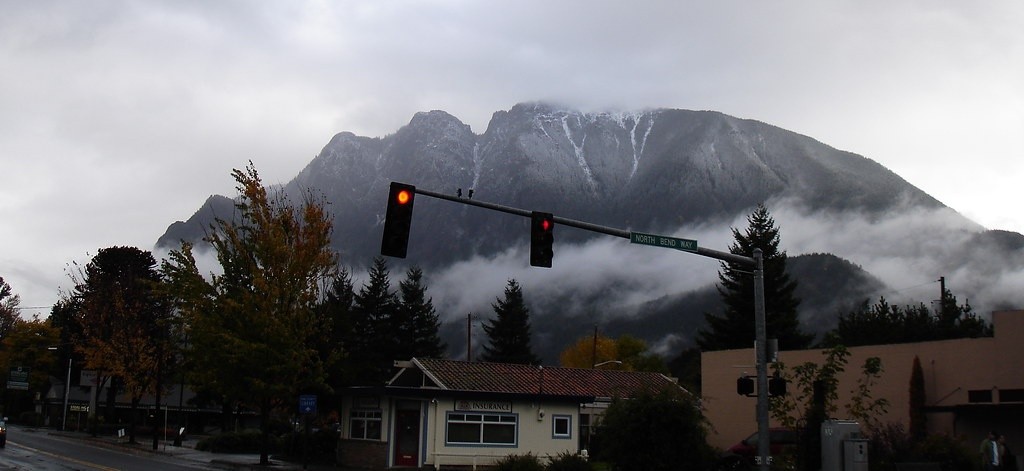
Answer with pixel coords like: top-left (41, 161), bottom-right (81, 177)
top-left (528, 209), bottom-right (555, 268)
top-left (768, 378), bottom-right (787, 396)
top-left (380, 180), bottom-right (416, 259)
top-left (736, 377), bottom-right (755, 395)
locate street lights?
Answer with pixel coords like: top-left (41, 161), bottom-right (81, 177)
top-left (48, 344), bottom-right (72, 431)
top-left (591, 323), bottom-right (623, 370)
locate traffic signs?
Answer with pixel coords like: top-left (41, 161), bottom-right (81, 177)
top-left (299, 394), bottom-right (317, 415)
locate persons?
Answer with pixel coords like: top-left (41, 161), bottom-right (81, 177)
top-left (994, 432), bottom-right (1013, 471)
top-left (979, 431), bottom-right (1004, 471)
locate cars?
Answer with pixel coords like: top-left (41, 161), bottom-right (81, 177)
top-left (0, 411), bottom-right (9, 448)
top-left (721, 426), bottom-right (806, 471)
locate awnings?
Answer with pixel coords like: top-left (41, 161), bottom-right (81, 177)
top-left (923, 401), bottom-right (1024, 415)
